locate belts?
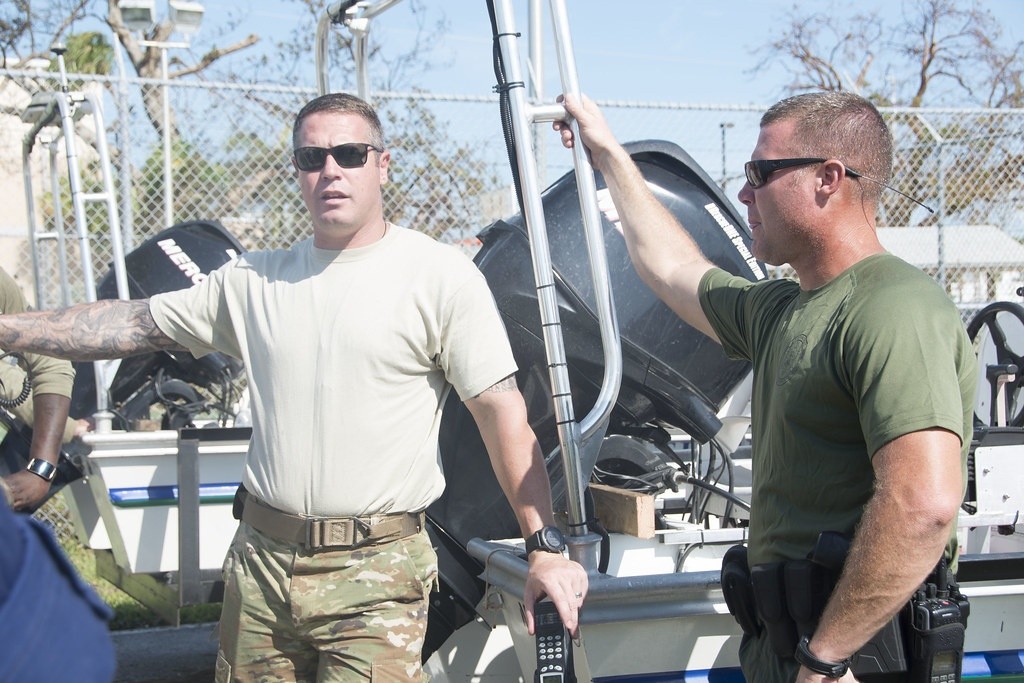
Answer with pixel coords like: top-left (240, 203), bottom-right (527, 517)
top-left (242, 494), bottom-right (420, 548)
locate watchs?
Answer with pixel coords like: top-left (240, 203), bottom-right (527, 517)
top-left (25, 456), bottom-right (59, 483)
top-left (795, 635), bottom-right (851, 679)
top-left (525, 526), bottom-right (567, 561)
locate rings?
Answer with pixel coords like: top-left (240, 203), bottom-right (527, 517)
top-left (575, 591), bottom-right (582, 598)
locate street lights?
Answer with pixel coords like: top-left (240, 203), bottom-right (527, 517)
top-left (116, 0), bottom-right (206, 231)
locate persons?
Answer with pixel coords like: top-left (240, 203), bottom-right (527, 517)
top-left (2, 259), bottom-right (107, 548)
top-left (555, 91), bottom-right (979, 683)
top-left (2, 94), bottom-right (590, 683)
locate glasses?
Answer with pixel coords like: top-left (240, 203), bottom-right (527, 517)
top-left (745, 158), bottom-right (935, 214)
top-left (294, 143), bottom-right (382, 171)
